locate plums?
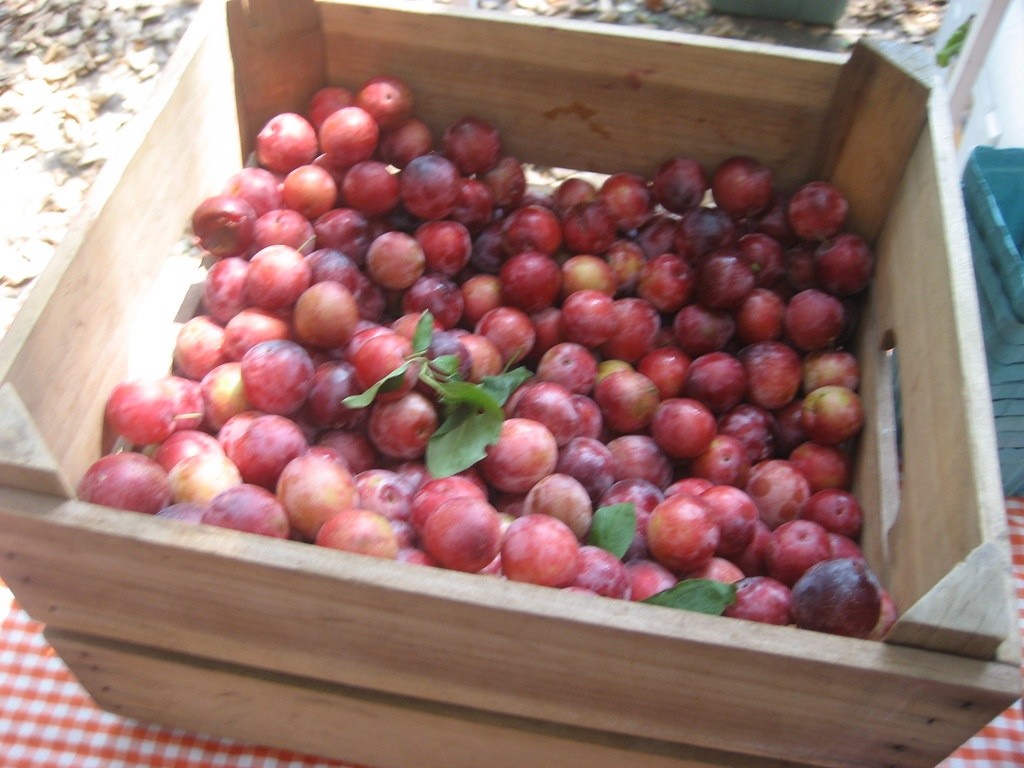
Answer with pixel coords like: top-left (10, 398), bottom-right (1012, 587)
top-left (73, 80), bottom-right (898, 644)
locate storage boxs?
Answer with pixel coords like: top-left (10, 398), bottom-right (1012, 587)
top-left (0, 0), bottom-right (1024, 768)
top-left (958, 147), bottom-right (1024, 499)
